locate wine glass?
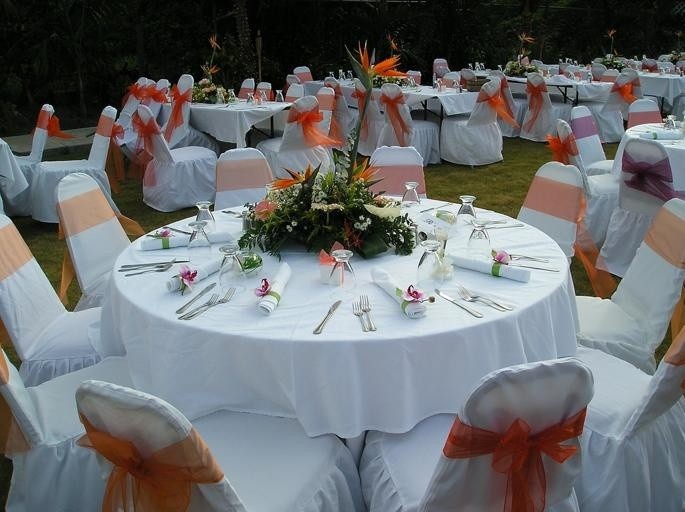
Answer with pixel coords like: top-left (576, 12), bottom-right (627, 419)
top-left (397, 76), bottom-right (417, 90)
top-left (465, 61), bottom-right (503, 73)
top-left (329, 72), bottom-right (334, 78)
top-left (258, 89), bottom-right (268, 104)
top-left (216, 91), bottom-right (225, 106)
top-left (247, 92), bottom-right (255, 107)
top-left (194, 201), bottom-right (217, 232)
top-left (414, 240), bottom-right (444, 293)
top-left (229, 89), bottom-right (236, 103)
top-left (664, 115), bottom-right (676, 130)
top-left (339, 72), bottom-right (345, 82)
top-left (218, 244), bottom-right (247, 293)
top-left (467, 219), bottom-right (492, 261)
top-left (347, 71), bottom-right (353, 79)
top-left (402, 181), bottom-right (421, 213)
top-left (275, 90), bottom-right (284, 105)
top-left (187, 221), bottom-right (213, 266)
top-left (456, 195), bottom-right (476, 229)
top-left (329, 249), bottom-right (358, 302)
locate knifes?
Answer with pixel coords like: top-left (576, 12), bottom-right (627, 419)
top-left (433, 287), bottom-right (482, 319)
top-left (175, 282), bottom-right (217, 314)
top-left (162, 226), bottom-right (192, 237)
top-left (121, 259), bottom-right (191, 268)
top-left (313, 300), bottom-right (342, 334)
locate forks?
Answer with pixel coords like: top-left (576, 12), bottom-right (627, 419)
top-left (361, 295), bottom-right (376, 331)
top-left (117, 257), bottom-right (177, 272)
top-left (124, 264), bottom-right (174, 277)
top-left (460, 285), bottom-right (513, 311)
top-left (351, 302), bottom-right (369, 333)
top-left (178, 293), bottom-right (219, 320)
top-left (457, 289), bottom-right (505, 314)
top-left (183, 287), bottom-right (237, 322)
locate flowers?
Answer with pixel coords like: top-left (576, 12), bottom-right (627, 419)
top-left (599, 29), bottom-right (624, 71)
top-left (670, 29), bottom-right (681, 59)
top-left (375, 32), bottom-right (405, 88)
top-left (191, 34), bottom-right (227, 105)
top-left (233, 40), bottom-right (418, 254)
top-left (506, 31), bottom-right (535, 77)
